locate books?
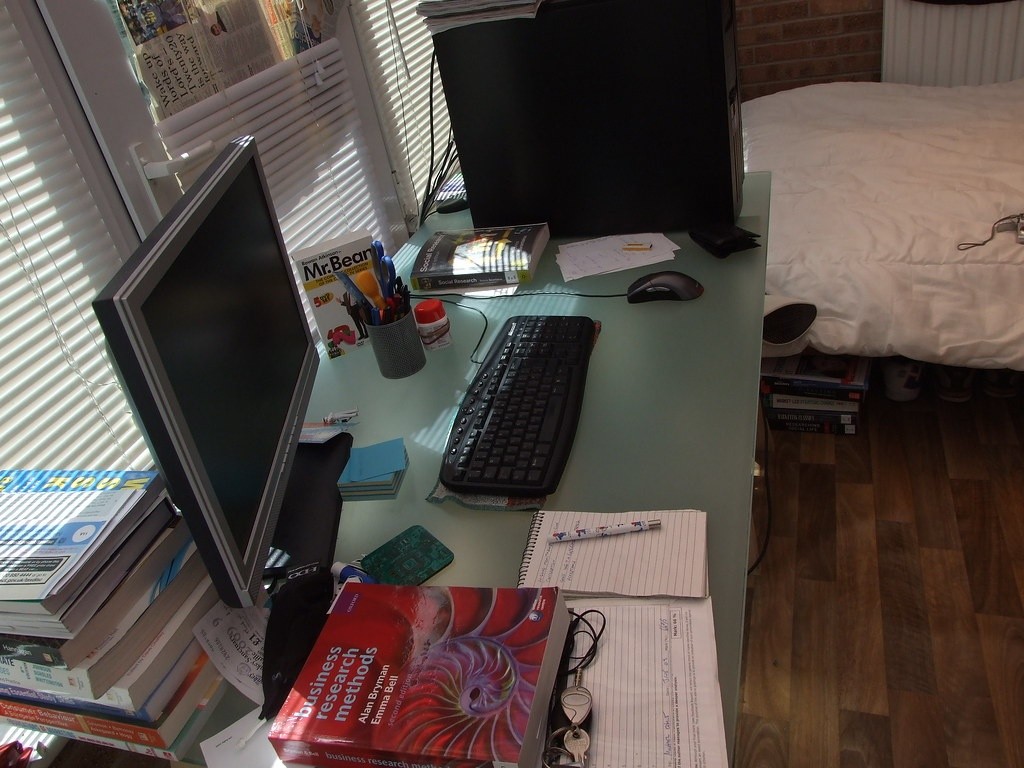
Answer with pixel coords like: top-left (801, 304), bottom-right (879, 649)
top-left (410, 222), bottom-right (551, 291)
top-left (0, 467), bottom-right (221, 763)
top-left (759, 347), bottom-right (873, 435)
top-left (266, 508), bottom-right (710, 768)
top-left (415, 0), bottom-right (542, 35)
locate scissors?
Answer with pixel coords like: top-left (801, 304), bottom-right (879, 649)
top-left (369, 240), bottom-right (396, 303)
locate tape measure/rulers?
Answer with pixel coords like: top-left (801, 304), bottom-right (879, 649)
top-left (331, 269), bottom-right (376, 320)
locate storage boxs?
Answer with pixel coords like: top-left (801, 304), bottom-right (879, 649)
top-left (289, 227), bottom-right (388, 360)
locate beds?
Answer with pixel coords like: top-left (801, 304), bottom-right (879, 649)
top-left (738, 81), bottom-right (1024, 372)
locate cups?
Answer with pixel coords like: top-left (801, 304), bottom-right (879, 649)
top-left (363, 305), bottom-right (426, 379)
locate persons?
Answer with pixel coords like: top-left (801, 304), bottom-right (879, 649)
top-left (211, 11), bottom-right (228, 37)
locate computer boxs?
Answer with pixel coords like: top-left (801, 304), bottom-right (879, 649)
top-left (431, 0), bottom-right (744, 242)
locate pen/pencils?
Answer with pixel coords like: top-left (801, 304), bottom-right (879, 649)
top-left (370, 275), bottom-right (412, 326)
top-left (544, 517), bottom-right (661, 545)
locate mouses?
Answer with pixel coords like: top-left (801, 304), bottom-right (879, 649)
top-left (626, 272), bottom-right (704, 304)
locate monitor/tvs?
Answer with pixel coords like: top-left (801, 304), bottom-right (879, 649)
top-left (92, 134), bottom-right (353, 610)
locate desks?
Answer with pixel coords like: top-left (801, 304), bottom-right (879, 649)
top-left (166, 172), bottom-right (773, 768)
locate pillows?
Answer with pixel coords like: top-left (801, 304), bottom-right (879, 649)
top-left (880, 0), bottom-right (1024, 86)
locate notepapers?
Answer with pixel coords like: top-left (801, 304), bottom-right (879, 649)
top-left (336, 437), bottom-right (410, 502)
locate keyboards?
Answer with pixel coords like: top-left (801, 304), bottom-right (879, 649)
top-left (442, 315), bottom-right (595, 495)
